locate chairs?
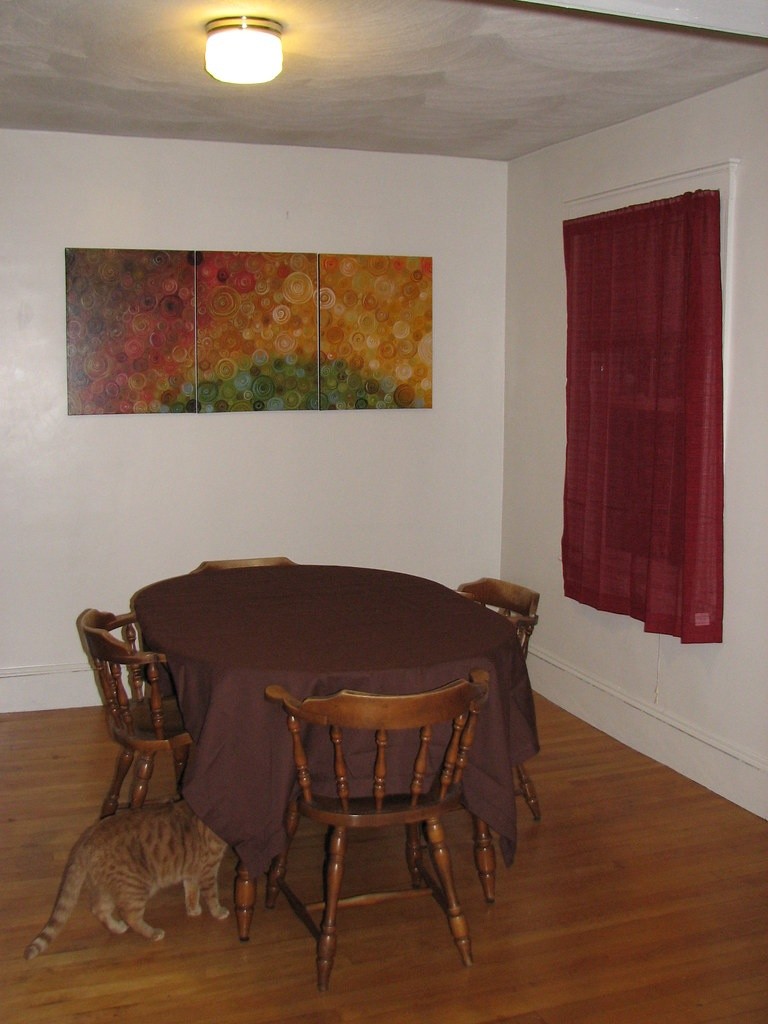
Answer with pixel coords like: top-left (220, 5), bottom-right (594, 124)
top-left (78, 608), bottom-right (194, 820)
top-left (455, 578), bottom-right (543, 820)
top-left (265, 669), bottom-right (491, 995)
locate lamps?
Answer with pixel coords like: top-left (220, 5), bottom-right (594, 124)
top-left (205, 16), bottom-right (283, 84)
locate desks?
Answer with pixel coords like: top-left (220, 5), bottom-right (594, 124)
top-left (134, 565), bottom-right (540, 943)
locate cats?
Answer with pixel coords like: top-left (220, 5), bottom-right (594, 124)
top-left (22, 797), bottom-right (231, 962)
